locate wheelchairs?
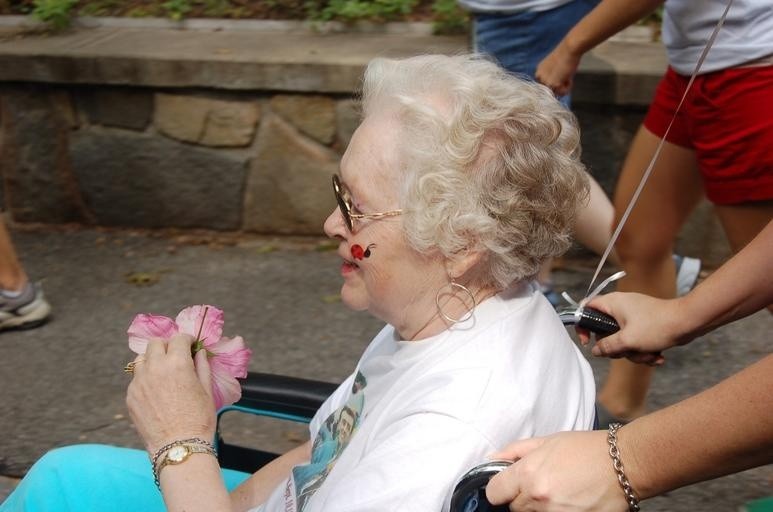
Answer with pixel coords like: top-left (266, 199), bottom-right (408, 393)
top-left (212, 309), bottom-right (620, 512)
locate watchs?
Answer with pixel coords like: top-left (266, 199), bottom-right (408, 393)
top-left (158, 443), bottom-right (217, 492)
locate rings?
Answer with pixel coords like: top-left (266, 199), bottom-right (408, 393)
top-left (125, 360), bottom-right (144, 376)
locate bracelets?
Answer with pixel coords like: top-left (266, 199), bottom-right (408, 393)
top-left (608, 423), bottom-right (641, 511)
top-left (153, 437), bottom-right (217, 472)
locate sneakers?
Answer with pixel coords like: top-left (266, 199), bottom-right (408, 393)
top-left (1, 283), bottom-right (51, 330)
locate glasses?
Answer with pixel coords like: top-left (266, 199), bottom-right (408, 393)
top-left (331, 174), bottom-right (407, 232)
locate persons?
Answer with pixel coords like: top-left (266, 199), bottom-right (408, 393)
top-left (0, 210), bottom-right (54, 333)
top-left (486, 217), bottom-right (773, 512)
top-left (534, 0), bottom-right (773, 430)
top-left (463, 0), bottom-right (702, 311)
top-left (0, 52), bottom-right (597, 512)
